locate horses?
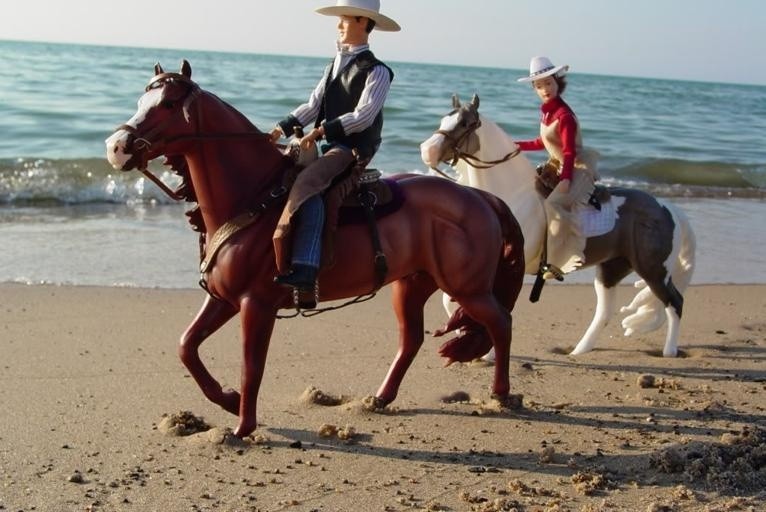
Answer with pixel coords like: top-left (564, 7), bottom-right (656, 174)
top-left (102, 58), bottom-right (526, 441)
top-left (418, 89), bottom-right (699, 360)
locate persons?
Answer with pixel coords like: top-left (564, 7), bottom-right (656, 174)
top-left (287, 2), bottom-right (623, 320)
